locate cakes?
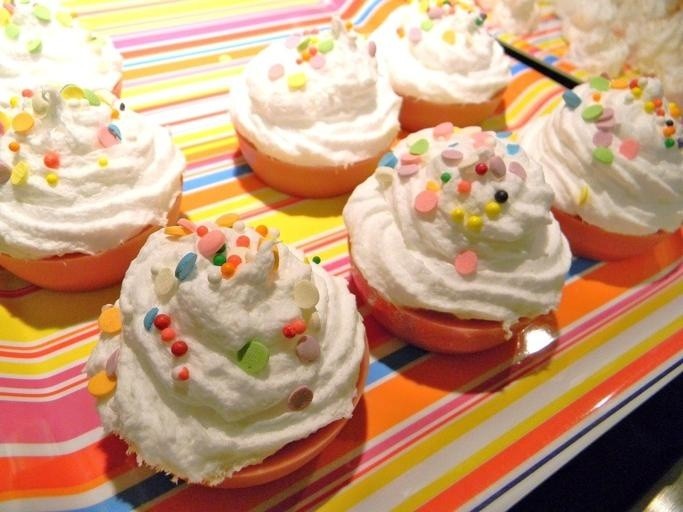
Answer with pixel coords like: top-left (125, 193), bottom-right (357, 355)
top-left (0, 85), bottom-right (187, 292)
top-left (88, 215), bottom-right (369, 490)
top-left (344, 123), bottom-right (572, 355)
top-left (520, 75), bottom-right (682, 261)
top-left (367, 0), bottom-right (510, 131)
top-left (229, 18), bottom-right (404, 197)
top-left (0, 0), bottom-right (123, 100)
